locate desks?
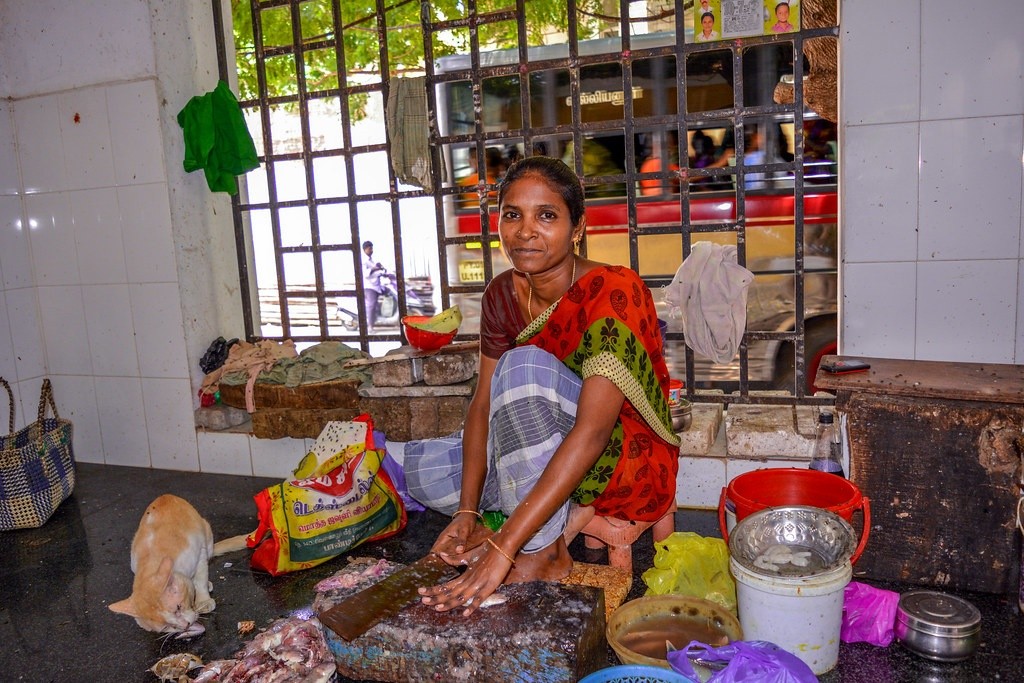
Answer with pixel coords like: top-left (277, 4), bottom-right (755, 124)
top-left (813, 354), bottom-right (1024, 600)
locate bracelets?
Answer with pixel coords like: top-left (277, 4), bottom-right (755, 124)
top-left (488, 539), bottom-right (516, 564)
top-left (451, 510), bottom-right (485, 522)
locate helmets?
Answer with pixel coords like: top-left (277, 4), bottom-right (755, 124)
top-left (401, 304), bottom-right (463, 353)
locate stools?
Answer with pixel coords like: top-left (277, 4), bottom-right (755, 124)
top-left (578, 499), bottom-right (676, 572)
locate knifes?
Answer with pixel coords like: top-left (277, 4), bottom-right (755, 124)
top-left (317, 523), bottom-right (494, 641)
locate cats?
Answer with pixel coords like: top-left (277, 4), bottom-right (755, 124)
top-left (106, 494), bottom-right (216, 634)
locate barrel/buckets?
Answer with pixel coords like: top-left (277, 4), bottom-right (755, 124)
top-left (718, 468), bottom-right (870, 560)
top-left (727, 562), bottom-right (859, 675)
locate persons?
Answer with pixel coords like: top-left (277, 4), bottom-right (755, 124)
top-left (461, 120), bottom-right (838, 207)
top-left (418, 155), bottom-right (682, 616)
top-left (361, 240), bottom-right (387, 333)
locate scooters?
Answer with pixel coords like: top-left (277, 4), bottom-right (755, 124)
top-left (334, 262), bottom-right (430, 332)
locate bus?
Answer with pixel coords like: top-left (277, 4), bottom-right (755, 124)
top-left (423, 20), bottom-right (839, 393)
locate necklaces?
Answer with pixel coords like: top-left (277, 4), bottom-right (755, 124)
top-left (527, 257), bottom-right (576, 322)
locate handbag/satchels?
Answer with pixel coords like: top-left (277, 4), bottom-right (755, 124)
top-left (0, 376), bottom-right (76, 532)
top-left (641, 531), bottom-right (739, 619)
top-left (666, 640), bottom-right (819, 683)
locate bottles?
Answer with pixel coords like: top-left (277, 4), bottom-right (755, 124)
top-left (809, 412), bottom-right (846, 478)
top-left (667, 379), bottom-right (684, 405)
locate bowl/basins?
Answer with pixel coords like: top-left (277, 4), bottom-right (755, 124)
top-left (895, 591), bottom-right (982, 661)
top-left (726, 504), bottom-right (858, 578)
top-left (667, 397), bottom-right (692, 432)
top-left (607, 594), bottom-right (743, 667)
top-left (401, 305), bottom-right (463, 351)
